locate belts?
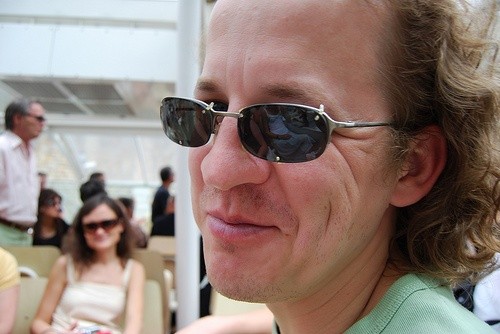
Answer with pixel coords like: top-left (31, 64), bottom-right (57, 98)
top-left (0, 218), bottom-right (29, 232)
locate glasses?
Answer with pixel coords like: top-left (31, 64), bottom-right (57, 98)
top-left (23, 112), bottom-right (44, 121)
top-left (82, 216), bottom-right (119, 233)
top-left (159, 95), bottom-right (395, 164)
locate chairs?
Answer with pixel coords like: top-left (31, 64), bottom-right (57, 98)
top-left (11, 236), bottom-right (176, 334)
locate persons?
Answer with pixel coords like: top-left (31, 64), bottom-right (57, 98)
top-left (0, 96), bottom-right (46, 247)
top-left (33, 188), bottom-right (68, 248)
top-left (152, 167), bottom-right (175, 236)
top-left (80, 172), bottom-right (147, 248)
top-left (30, 193), bottom-right (145, 334)
top-left (175, 0), bottom-right (500, 334)
top-left (239, 105), bottom-right (321, 162)
top-left (0, 246), bottom-right (21, 334)
